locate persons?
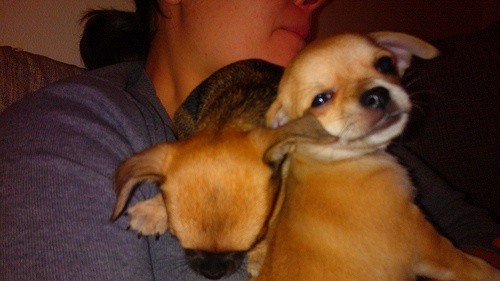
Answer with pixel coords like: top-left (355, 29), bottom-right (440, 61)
top-left (0, 0), bottom-right (324, 281)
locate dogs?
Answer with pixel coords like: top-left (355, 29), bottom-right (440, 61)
top-left (109, 57), bottom-right (339, 281)
top-left (254, 30), bottom-right (500, 281)
